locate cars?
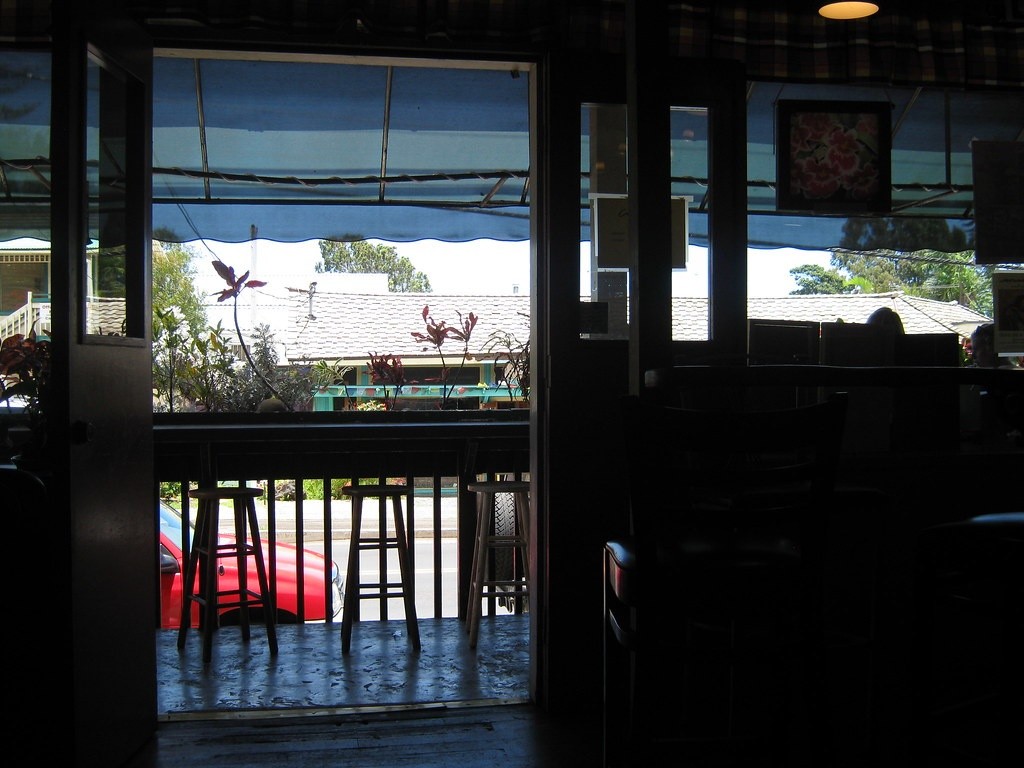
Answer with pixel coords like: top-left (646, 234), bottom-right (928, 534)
top-left (160, 498), bottom-right (344, 633)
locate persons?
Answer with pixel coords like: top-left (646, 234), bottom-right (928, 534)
top-left (868, 307), bottom-right (904, 334)
top-left (971, 324), bottom-right (1014, 367)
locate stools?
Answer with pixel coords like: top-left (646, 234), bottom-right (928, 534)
top-left (177, 487), bottom-right (279, 662)
top-left (467, 481), bottom-right (528, 647)
top-left (340, 485), bottom-right (421, 650)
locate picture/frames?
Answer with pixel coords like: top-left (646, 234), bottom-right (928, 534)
top-left (773, 98), bottom-right (891, 215)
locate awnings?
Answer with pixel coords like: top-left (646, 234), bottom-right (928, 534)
top-left (0, 49), bottom-right (1024, 250)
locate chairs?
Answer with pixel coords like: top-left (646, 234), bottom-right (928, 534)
top-left (597, 404), bottom-right (1024, 768)
